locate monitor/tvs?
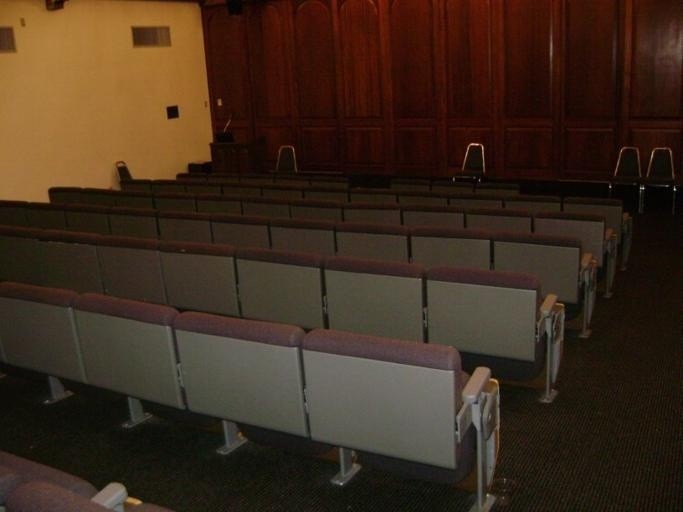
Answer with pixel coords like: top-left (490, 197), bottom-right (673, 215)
top-left (215, 133), bottom-right (234, 142)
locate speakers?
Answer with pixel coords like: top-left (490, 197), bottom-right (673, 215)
top-left (46, 2), bottom-right (64, 10)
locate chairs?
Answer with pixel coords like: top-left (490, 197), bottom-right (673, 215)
top-left (607, 144), bottom-right (679, 218)
top-left (0, 142), bottom-right (636, 512)
